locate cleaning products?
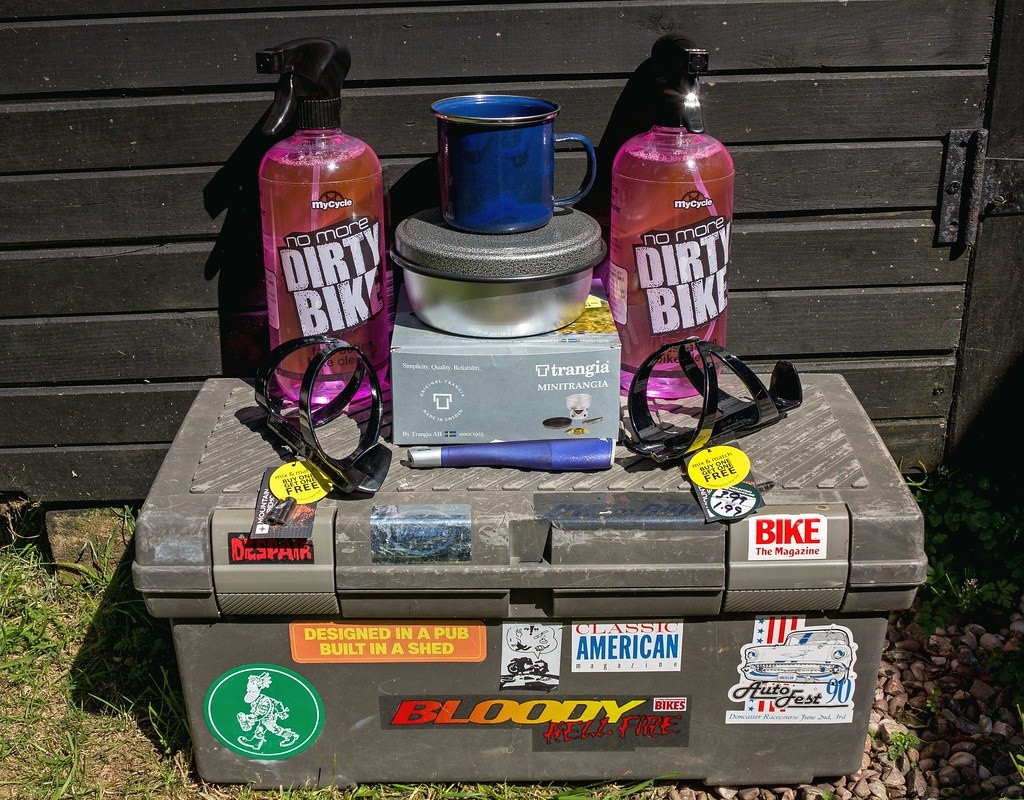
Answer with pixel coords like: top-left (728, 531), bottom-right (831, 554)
top-left (607, 33), bottom-right (736, 402)
top-left (253, 36), bottom-right (393, 407)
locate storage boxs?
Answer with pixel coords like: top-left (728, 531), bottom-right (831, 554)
top-left (131, 374), bottom-right (931, 790)
top-left (389, 282), bottom-right (620, 444)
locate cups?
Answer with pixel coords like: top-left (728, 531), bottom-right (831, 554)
top-left (431, 94), bottom-right (598, 233)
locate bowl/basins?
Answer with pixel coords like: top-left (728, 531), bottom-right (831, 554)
top-left (388, 208), bottom-right (609, 340)
